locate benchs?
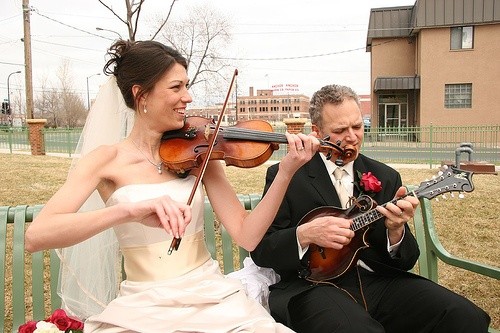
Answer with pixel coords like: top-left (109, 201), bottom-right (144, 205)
top-left (0, 184), bottom-right (500, 333)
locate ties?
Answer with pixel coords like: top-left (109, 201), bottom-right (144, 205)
top-left (332, 167), bottom-right (353, 209)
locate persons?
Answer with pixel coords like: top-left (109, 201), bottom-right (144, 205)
top-left (25, 39), bottom-right (320, 333)
top-left (251, 85), bottom-right (491, 333)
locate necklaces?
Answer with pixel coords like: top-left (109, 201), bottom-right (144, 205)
top-left (128, 134), bottom-right (163, 175)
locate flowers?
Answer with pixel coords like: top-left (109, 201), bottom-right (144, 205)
top-left (351, 169), bottom-right (383, 196)
top-left (18, 309), bottom-right (84, 333)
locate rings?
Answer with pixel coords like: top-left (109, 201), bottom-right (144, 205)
top-left (297, 147), bottom-right (304, 151)
top-left (398, 209), bottom-right (403, 216)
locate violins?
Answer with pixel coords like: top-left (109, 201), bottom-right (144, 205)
top-left (158, 115), bottom-right (358, 175)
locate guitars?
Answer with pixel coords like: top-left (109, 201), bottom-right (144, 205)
top-left (296, 164), bottom-right (475, 282)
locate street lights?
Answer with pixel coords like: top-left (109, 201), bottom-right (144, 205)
top-left (7, 70), bottom-right (21, 118)
top-left (87, 73), bottom-right (101, 112)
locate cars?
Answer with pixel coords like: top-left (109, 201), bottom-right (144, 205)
top-left (363, 118), bottom-right (371, 132)
